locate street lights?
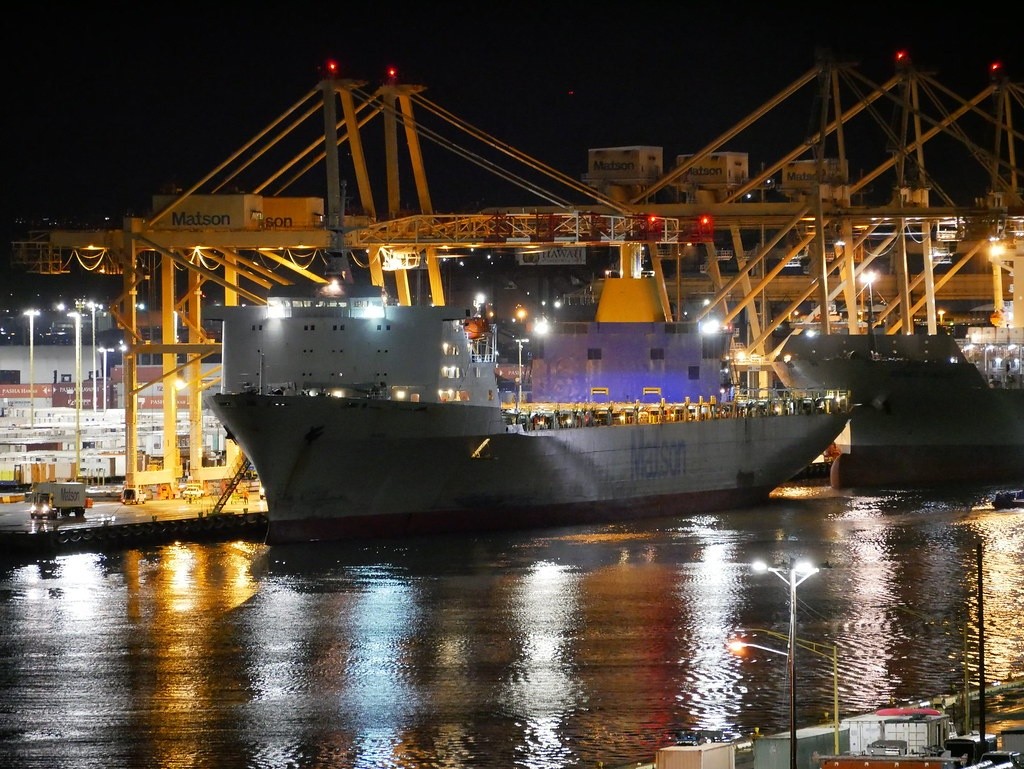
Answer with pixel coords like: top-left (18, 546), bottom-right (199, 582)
top-left (87, 301), bottom-right (103, 414)
top-left (24, 309), bottom-right (40, 429)
top-left (97, 346), bottom-right (114, 412)
top-left (66, 310), bottom-right (83, 473)
top-left (727, 561), bottom-right (840, 769)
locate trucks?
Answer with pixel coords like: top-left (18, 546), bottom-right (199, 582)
top-left (122, 487), bottom-right (147, 505)
top-left (31, 482), bottom-right (86, 519)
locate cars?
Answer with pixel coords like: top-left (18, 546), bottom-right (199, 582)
top-left (184, 487), bottom-right (200, 500)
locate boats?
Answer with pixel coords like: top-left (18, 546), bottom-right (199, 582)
top-left (771, 326), bottom-right (1024, 490)
top-left (202, 281), bottom-right (851, 544)
